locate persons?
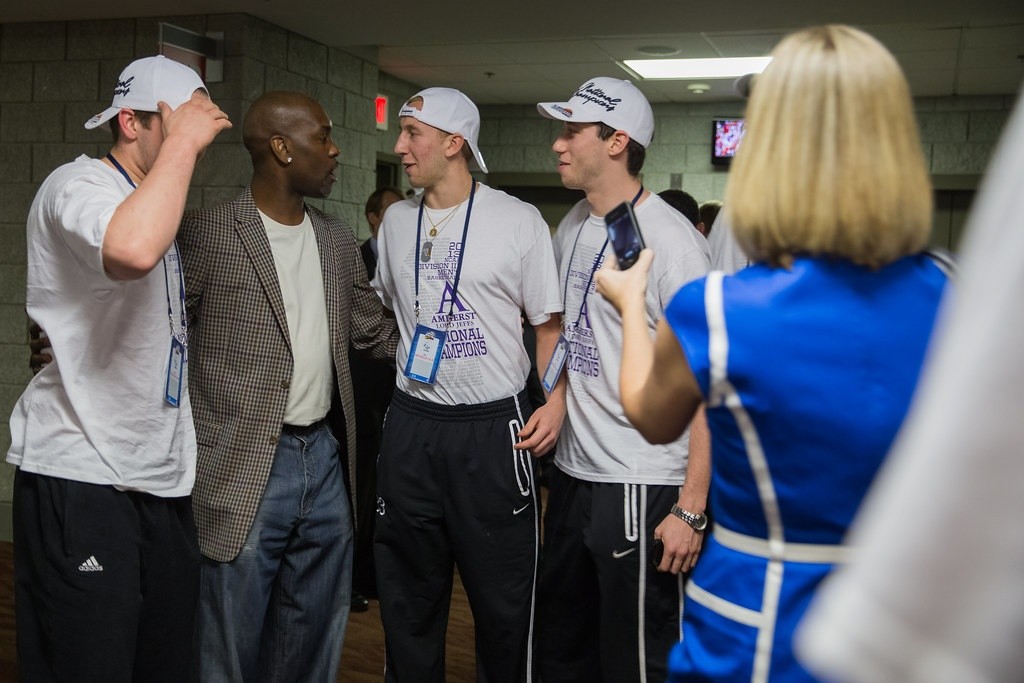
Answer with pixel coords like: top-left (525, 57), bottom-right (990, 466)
top-left (352, 69), bottom-right (1018, 681)
top-left (31, 90), bottom-right (401, 681)
top-left (2, 53), bottom-right (233, 679)
top-left (594, 19), bottom-right (969, 679)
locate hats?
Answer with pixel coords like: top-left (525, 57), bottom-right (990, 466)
top-left (537, 77), bottom-right (654, 150)
top-left (84, 55), bottom-right (210, 134)
top-left (399, 87), bottom-right (488, 174)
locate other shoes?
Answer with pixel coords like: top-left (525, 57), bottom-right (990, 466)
top-left (350, 585), bottom-right (368, 611)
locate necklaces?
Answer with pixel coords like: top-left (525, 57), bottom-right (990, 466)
top-left (417, 195), bottom-right (463, 262)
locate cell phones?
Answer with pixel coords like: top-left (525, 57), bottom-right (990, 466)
top-left (604, 200), bottom-right (647, 271)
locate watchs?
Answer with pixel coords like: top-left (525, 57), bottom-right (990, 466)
top-left (670, 502), bottom-right (708, 532)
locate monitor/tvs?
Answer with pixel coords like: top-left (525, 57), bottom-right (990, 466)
top-left (711, 119), bottom-right (744, 165)
